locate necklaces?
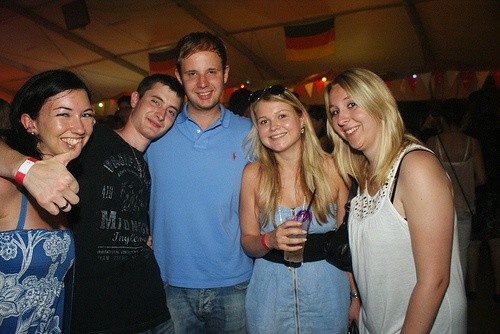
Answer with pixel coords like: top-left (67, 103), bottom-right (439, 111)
top-left (128, 143), bottom-right (146, 177)
top-left (279, 192), bottom-right (306, 269)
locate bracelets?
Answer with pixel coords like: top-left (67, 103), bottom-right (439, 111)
top-left (352, 293), bottom-right (360, 299)
top-left (261, 234), bottom-right (271, 250)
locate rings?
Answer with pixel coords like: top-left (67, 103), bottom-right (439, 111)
top-left (60, 203), bottom-right (68, 211)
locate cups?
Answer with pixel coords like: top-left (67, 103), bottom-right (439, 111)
top-left (282, 216), bottom-right (311, 263)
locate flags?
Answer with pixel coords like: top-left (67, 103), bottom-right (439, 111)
top-left (284, 17), bottom-right (336, 62)
top-left (148, 48), bottom-right (176, 77)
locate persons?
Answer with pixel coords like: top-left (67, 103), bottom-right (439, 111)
top-left (0, 69), bottom-right (94, 334)
top-left (229, 89), bottom-right (255, 118)
top-left (141, 32), bottom-right (259, 334)
top-left (405, 101), bottom-right (436, 150)
top-left (465, 91), bottom-right (500, 303)
top-left (0, 73), bottom-right (187, 334)
top-left (238, 86), bottom-right (358, 334)
top-left (117, 96), bottom-right (132, 127)
top-left (324, 68), bottom-right (467, 334)
top-left (426, 99), bottom-right (486, 282)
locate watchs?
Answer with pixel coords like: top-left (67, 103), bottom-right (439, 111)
top-left (15, 158), bottom-right (36, 186)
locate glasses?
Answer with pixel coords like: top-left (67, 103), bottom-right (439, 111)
top-left (249, 84), bottom-right (286, 102)
